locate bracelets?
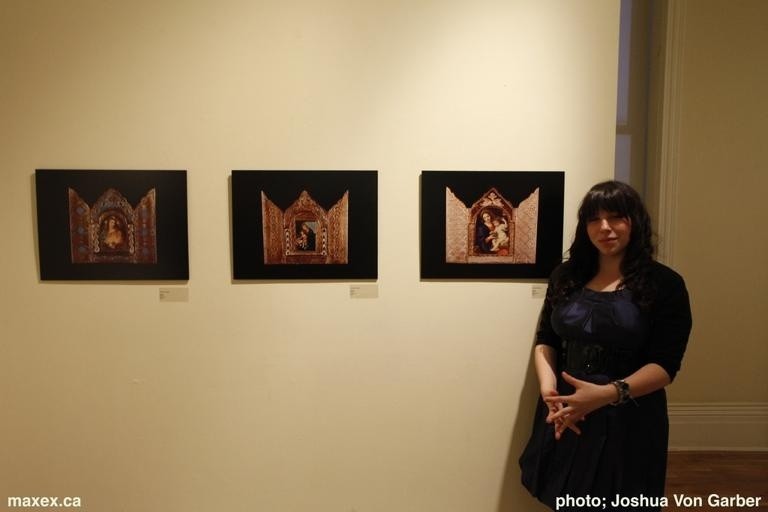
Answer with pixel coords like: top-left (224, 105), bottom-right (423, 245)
top-left (610, 380), bottom-right (630, 409)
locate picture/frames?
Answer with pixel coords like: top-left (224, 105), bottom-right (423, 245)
top-left (420, 171), bottom-right (565, 280)
top-left (34, 168), bottom-right (190, 280)
top-left (231, 169), bottom-right (378, 280)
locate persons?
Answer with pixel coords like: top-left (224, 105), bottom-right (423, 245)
top-left (519, 181), bottom-right (693, 511)
top-left (98, 216), bottom-right (125, 252)
top-left (298, 224), bottom-right (315, 250)
top-left (477, 210), bottom-right (509, 254)
